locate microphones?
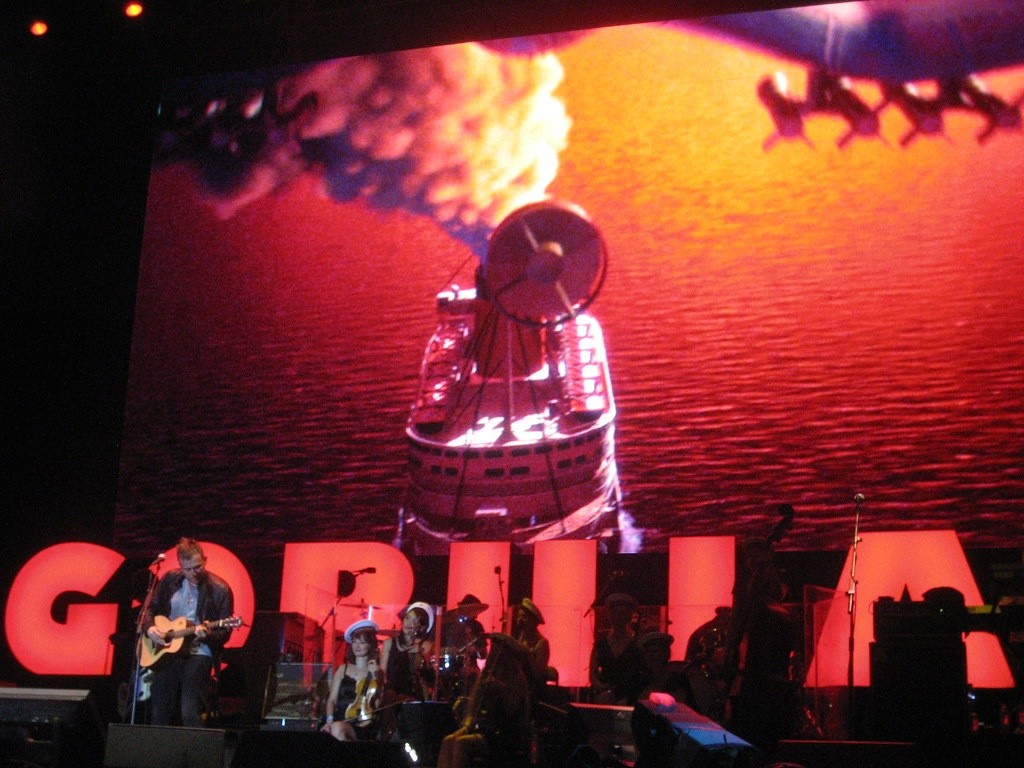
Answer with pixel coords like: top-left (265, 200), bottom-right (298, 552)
top-left (152, 554), bottom-right (166, 565)
top-left (354, 568), bottom-right (377, 574)
top-left (854, 493), bottom-right (865, 504)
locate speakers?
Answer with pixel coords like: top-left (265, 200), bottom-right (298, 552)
top-left (103, 722), bottom-right (241, 768)
top-left (560, 702), bottom-right (637, 761)
top-left (229, 727), bottom-right (366, 768)
top-left (0, 685), bottom-right (108, 768)
top-left (870, 638), bottom-right (970, 750)
top-left (261, 664), bottom-right (331, 721)
top-left (341, 740), bottom-right (421, 768)
top-left (631, 701), bottom-right (763, 768)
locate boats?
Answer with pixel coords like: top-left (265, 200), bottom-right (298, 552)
top-left (402, 192), bottom-right (628, 557)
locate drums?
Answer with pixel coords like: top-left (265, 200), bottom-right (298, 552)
top-left (421, 655), bottom-right (461, 672)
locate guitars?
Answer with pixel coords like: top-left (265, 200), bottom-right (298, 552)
top-left (136, 615), bottom-right (243, 671)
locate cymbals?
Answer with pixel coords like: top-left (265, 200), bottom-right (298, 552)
top-left (443, 604), bottom-right (490, 624)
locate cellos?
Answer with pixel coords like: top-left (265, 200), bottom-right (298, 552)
top-left (701, 503), bottom-right (796, 756)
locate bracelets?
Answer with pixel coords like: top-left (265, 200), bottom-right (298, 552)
top-left (326, 715), bottom-right (334, 721)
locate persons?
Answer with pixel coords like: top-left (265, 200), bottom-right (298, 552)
top-left (142, 536), bottom-right (235, 728)
top-left (323, 593), bottom-right (731, 768)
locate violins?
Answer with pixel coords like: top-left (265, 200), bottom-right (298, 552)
top-left (344, 648), bottom-right (379, 728)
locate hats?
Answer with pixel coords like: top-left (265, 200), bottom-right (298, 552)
top-left (406, 602), bottom-right (434, 632)
top-left (606, 594), bottom-right (636, 606)
top-left (343, 619), bottom-right (377, 643)
top-left (445, 594), bottom-right (489, 623)
top-left (636, 632), bottom-right (674, 652)
top-left (738, 538), bottom-right (763, 563)
top-left (523, 598), bottom-right (543, 623)
